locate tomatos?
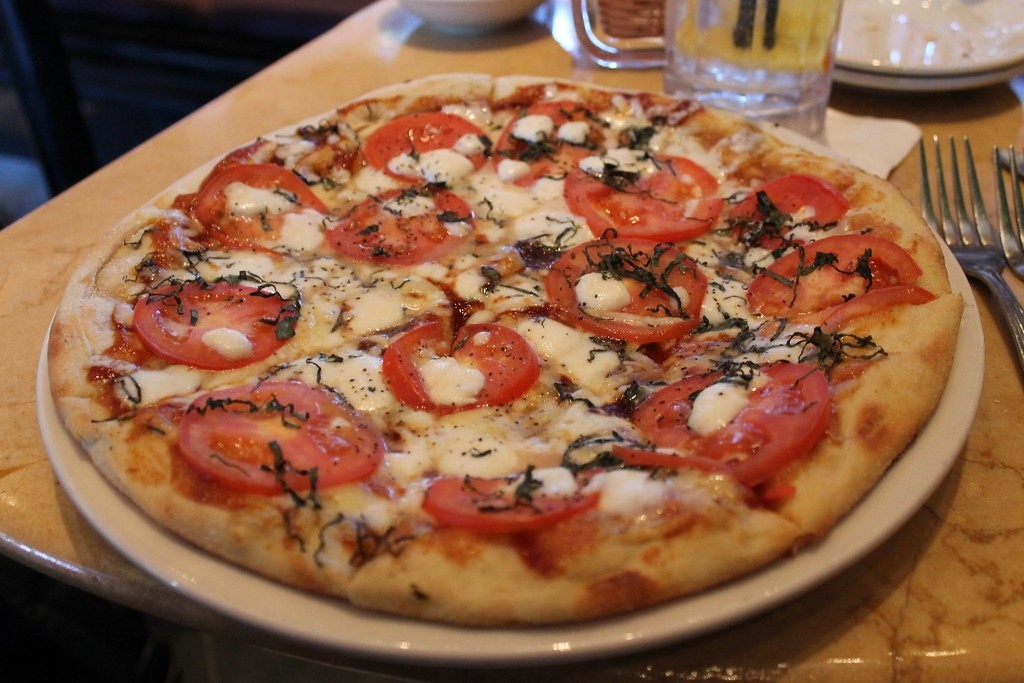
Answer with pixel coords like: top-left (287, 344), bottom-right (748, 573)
top-left (128, 100), bottom-right (938, 533)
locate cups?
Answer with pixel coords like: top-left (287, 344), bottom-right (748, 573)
top-left (567, 0), bottom-right (845, 140)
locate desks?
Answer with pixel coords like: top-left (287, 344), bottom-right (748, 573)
top-left (0, 0), bottom-right (1024, 683)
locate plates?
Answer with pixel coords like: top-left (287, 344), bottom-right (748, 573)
top-left (832, 0), bottom-right (1024, 91)
top-left (35, 70), bottom-right (985, 667)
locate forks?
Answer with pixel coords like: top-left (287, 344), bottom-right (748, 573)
top-left (916, 133), bottom-right (1024, 389)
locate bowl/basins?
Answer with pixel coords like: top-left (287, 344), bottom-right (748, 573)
top-left (394, 0), bottom-right (551, 37)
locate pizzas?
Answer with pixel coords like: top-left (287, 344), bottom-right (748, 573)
top-left (46, 72), bottom-right (966, 630)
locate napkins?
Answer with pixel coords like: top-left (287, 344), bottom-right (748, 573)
top-left (826, 106), bottom-right (922, 181)
